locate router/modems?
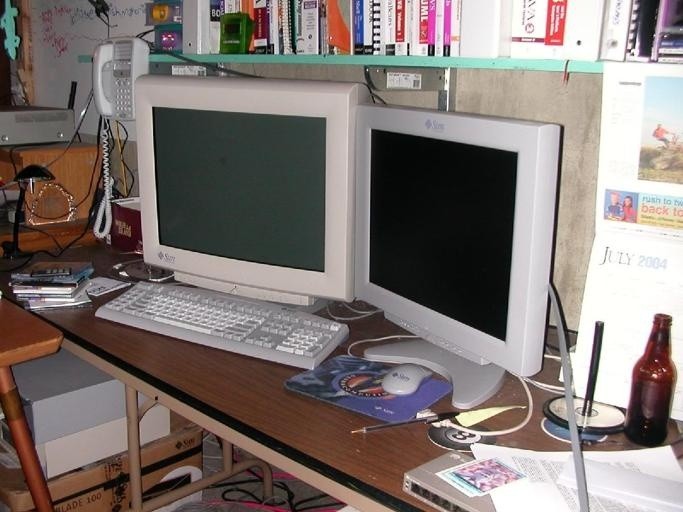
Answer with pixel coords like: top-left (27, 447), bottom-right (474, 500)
top-left (402, 450), bottom-right (497, 512)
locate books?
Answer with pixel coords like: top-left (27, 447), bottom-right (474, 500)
top-left (9, 261), bottom-right (94, 309)
top-left (209, 1), bottom-right (682, 62)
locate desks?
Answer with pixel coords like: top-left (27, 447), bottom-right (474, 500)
top-left (1, 244), bottom-right (682, 512)
top-left (0, 296), bottom-right (63, 512)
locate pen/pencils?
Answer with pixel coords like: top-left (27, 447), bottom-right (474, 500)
top-left (350, 412), bottom-right (459, 434)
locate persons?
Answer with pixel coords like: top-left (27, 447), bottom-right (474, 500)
top-left (606, 192), bottom-right (637, 223)
top-left (652, 124), bottom-right (670, 148)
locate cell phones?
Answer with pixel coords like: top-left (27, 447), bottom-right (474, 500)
top-left (31, 267), bottom-right (71, 277)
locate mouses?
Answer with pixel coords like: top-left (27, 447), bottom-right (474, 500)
top-left (382, 362), bottom-right (433, 396)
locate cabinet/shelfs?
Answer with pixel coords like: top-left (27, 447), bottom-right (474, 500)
top-left (0, 143), bottom-right (105, 258)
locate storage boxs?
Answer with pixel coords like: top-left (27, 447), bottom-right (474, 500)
top-left (0, 346), bottom-right (203, 512)
top-left (109, 196), bottom-right (143, 252)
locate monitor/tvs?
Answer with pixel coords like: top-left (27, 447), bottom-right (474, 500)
top-left (353, 101), bottom-right (562, 410)
top-left (134, 73), bottom-right (371, 307)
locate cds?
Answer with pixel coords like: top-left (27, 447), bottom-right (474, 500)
top-left (549, 396), bottom-right (625, 428)
top-left (125, 261), bottom-right (173, 280)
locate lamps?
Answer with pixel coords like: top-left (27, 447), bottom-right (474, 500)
top-left (0, 164), bottom-right (56, 256)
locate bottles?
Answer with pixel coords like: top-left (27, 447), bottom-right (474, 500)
top-left (621, 313), bottom-right (677, 447)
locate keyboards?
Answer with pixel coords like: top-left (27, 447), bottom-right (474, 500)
top-left (95, 280), bottom-right (350, 371)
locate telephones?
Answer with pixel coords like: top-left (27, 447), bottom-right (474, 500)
top-left (92, 37), bottom-right (150, 121)
